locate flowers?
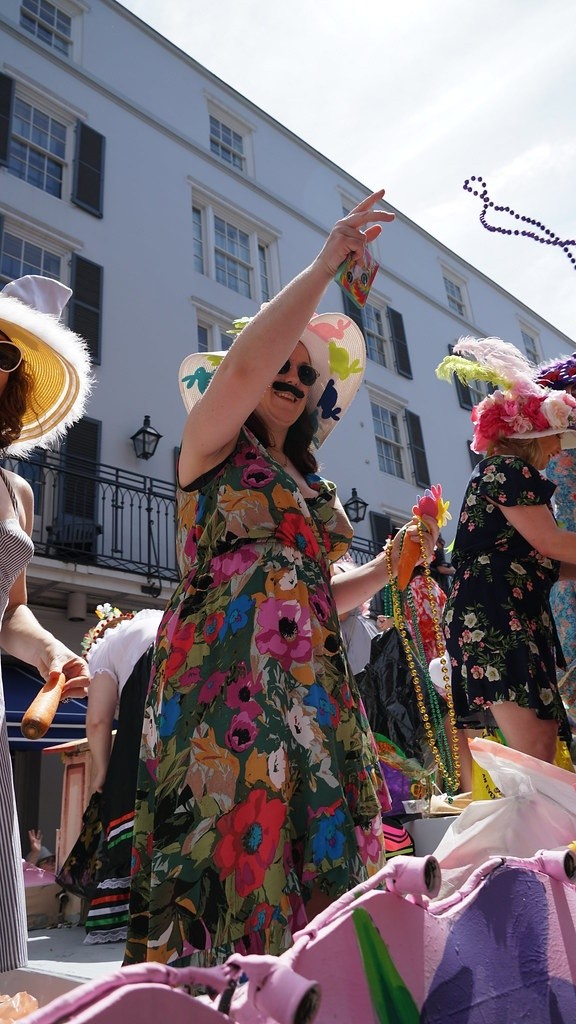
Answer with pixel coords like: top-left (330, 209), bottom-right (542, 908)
top-left (470, 392), bottom-right (576, 457)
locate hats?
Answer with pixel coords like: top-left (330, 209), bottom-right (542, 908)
top-left (179, 303), bottom-right (366, 450)
top-left (0, 275), bottom-right (98, 460)
top-left (429, 650), bottom-right (453, 691)
top-left (80, 604), bottom-right (137, 663)
top-left (436, 334), bottom-right (576, 450)
top-left (26, 847), bottom-right (55, 866)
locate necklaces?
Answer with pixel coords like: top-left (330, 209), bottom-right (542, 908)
top-left (382, 519), bottom-right (462, 804)
top-left (463, 176), bottom-right (576, 272)
top-left (269, 446), bottom-right (288, 467)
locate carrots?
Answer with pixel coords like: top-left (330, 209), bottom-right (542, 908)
top-left (21, 672), bottom-right (66, 739)
top-left (397, 524), bottom-right (421, 592)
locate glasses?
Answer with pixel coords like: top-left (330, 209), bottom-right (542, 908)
top-left (278, 360), bottom-right (321, 386)
top-left (38, 857), bottom-right (54, 865)
top-left (0, 340), bottom-right (23, 373)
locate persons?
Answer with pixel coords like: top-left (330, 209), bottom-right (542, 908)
top-left (440, 423), bottom-right (576, 763)
top-left (25, 829), bottom-right (56, 873)
top-left (532, 351), bottom-right (576, 734)
top-left (117, 191), bottom-right (442, 994)
top-left (58, 602), bottom-right (164, 947)
top-left (325, 546), bottom-right (471, 793)
top-left (0, 274), bottom-right (95, 978)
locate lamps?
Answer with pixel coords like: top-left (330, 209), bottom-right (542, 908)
top-left (130, 415), bottom-right (164, 461)
top-left (343, 488), bottom-right (369, 523)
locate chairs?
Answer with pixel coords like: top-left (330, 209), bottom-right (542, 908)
top-left (44, 521), bottom-right (106, 559)
